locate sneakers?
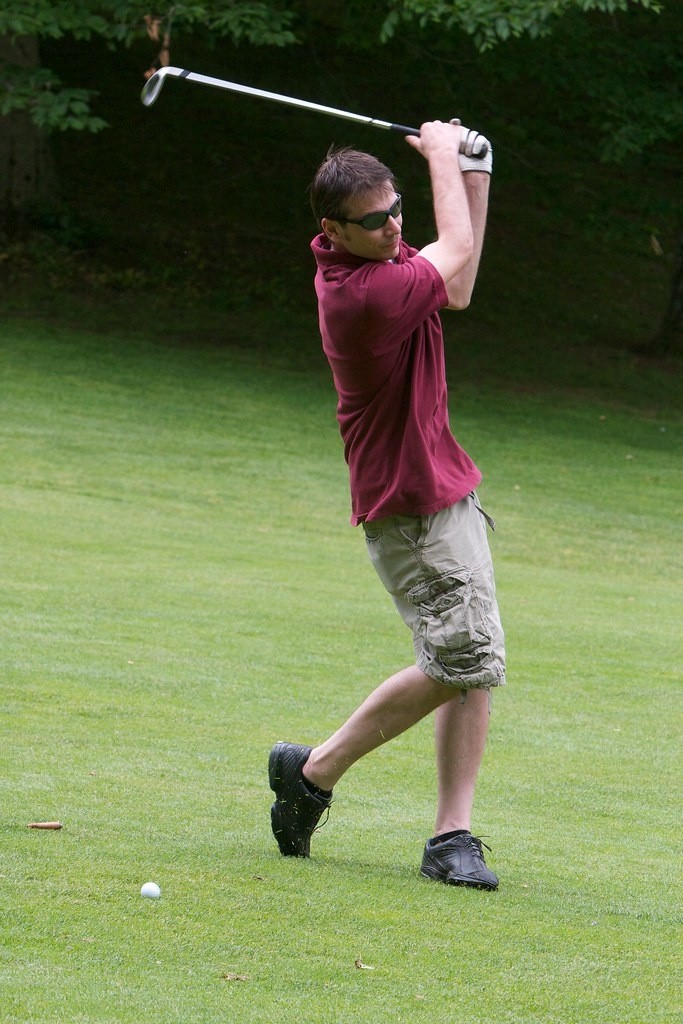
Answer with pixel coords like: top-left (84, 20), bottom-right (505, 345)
top-left (418, 834), bottom-right (499, 890)
top-left (267, 742), bottom-right (332, 857)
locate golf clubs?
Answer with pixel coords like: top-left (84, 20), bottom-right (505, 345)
top-left (140, 66), bottom-right (488, 160)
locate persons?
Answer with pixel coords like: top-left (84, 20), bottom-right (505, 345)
top-left (268, 116), bottom-right (506, 890)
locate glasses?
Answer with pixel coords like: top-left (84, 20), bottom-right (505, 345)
top-left (334, 194), bottom-right (402, 231)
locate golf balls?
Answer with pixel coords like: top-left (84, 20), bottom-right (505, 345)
top-left (140, 882), bottom-right (160, 898)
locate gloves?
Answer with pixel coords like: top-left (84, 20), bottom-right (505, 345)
top-left (449, 117), bottom-right (492, 174)
